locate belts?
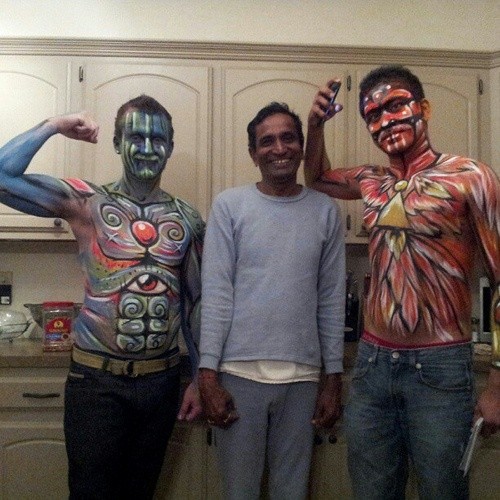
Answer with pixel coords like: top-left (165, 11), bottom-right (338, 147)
top-left (70, 346), bottom-right (181, 378)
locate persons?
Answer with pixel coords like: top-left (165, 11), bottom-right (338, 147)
top-left (304, 63), bottom-right (500, 500)
top-left (0, 96), bottom-right (207, 500)
top-left (197, 102), bottom-right (346, 500)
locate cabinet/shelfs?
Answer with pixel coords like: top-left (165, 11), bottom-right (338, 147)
top-left (0, 36), bottom-right (500, 240)
top-left (0, 367), bottom-right (500, 500)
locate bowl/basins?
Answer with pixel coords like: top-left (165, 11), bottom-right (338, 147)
top-left (23, 303), bottom-right (84, 330)
top-left (0, 320), bottom-right (33, 339)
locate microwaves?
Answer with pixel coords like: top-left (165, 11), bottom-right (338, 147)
top-left (479, 276), bottom-right (492, 344)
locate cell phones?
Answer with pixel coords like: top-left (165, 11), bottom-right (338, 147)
top-left (317, 81), bottom-right (341, 124)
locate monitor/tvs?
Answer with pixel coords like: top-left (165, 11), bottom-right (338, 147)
top-left (479, 277), bottom-right (493, 343)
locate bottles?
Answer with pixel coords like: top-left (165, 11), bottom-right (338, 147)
top-left (472, 317), bottom-right (480, 343)
top-left (42, 302), bottom-right (75, 352)
